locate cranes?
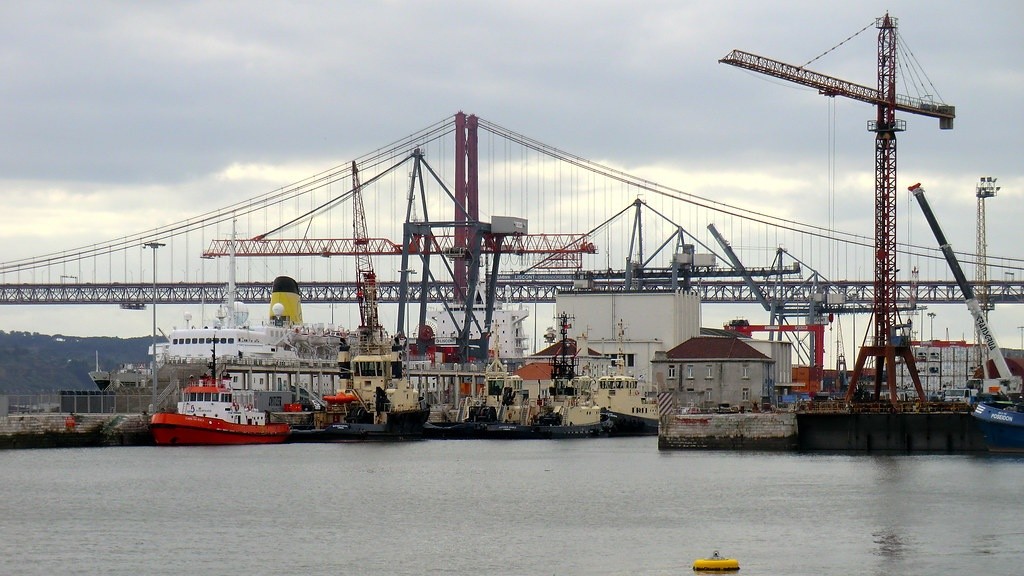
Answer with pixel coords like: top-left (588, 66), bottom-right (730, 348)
top-left (719, 12), bottom-right (956, 413)
top-left (205, 147), bottom-right (591, 358)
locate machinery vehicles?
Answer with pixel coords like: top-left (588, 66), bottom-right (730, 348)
top-left (908, 183), bottom-right (1022, 406)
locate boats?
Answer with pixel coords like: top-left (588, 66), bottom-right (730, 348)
top-left (151, 336), bottom-right (292, 445)
top-left (89, 349), bottom-right (149, 391)
top-left (281, 314), bottom-right (430, 441)
top-left (422, 313), bottom-right (614, 439)
top-left (600, 320), bottom-right (660, 437)
